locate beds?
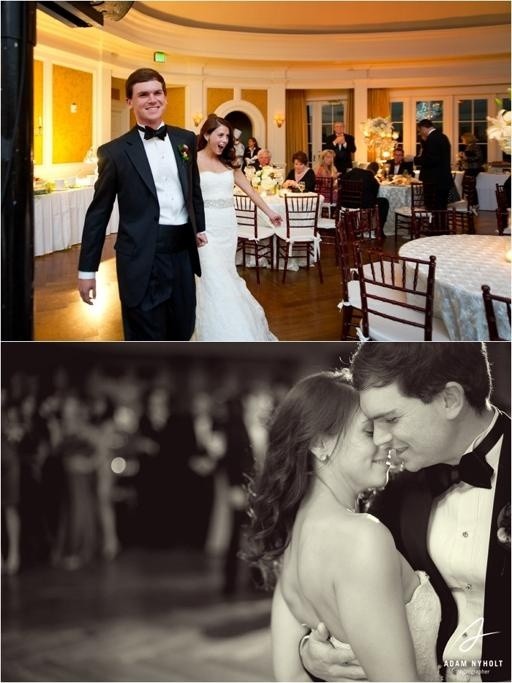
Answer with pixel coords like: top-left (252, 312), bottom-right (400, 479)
top-left (32, 184), bottom-right (120, 257)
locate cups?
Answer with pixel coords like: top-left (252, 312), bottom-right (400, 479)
top-left (54, 178), bottom-right (63, 189)
top-left (68, 176), bottom-right (76, 185)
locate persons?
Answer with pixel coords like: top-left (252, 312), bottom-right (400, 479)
top-left (196, 115), bottom-right (285, 342)
top-left (295, 342), bottom-right (509, 681)
top-left (0, 359), bottom-right (337, 575)
top-left (503, 174), bottom-right (511, 226)
top-left (459, 129), bottom-right (484, 215)
top-left (237, 367), bottom-right (443, 680)
top-left (231, 121), bottom-right (412, 240)
top-left (413, 119), bottom-right (460, 234)
top-left (79, 68), bottom-right (207, 341)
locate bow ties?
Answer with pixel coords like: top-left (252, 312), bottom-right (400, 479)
top-left (425, 452), bottom-right (494, 497)
top-left (144, 126), bottom-right (166, 140)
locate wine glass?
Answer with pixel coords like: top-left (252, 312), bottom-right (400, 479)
top-left (300, 181), bottom-right (305, 193)
top-left (244, 164), bottom-right (285, 195)
top-left (379, 165), bottom-right (415, 183)
